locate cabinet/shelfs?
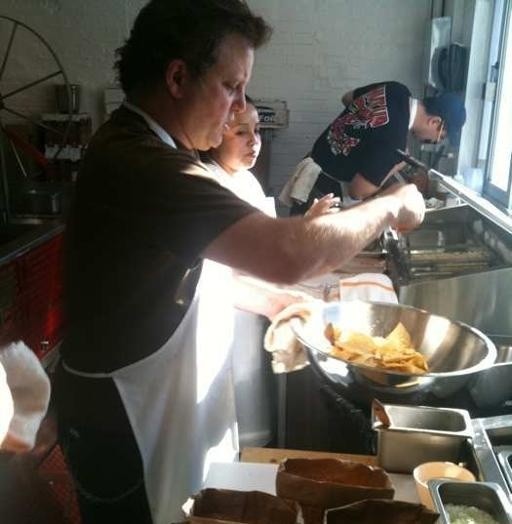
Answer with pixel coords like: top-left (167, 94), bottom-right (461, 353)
top-left (0, 230), bottom-right (68, 360)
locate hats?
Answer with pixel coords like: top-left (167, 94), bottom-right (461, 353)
top-left (434, 93), bottom-right (466, 146)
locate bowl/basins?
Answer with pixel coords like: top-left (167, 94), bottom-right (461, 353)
top-left (414, 461), bottom-right (476, 511)
top-left (292, 298), bottom-right (497, 415)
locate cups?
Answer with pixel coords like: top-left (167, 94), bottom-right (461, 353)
top-left (55, 84), bottom-right (81, 113)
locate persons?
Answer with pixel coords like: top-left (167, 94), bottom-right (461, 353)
top-left (56, 0), bottom-right (426, 524)
top-left (197, 94), bottom-right (278, 447)
top-left (289, 82), bottom-right (465, 216)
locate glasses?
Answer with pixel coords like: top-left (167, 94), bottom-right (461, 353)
top-left (436, 120), bottom-right (445, 144)
